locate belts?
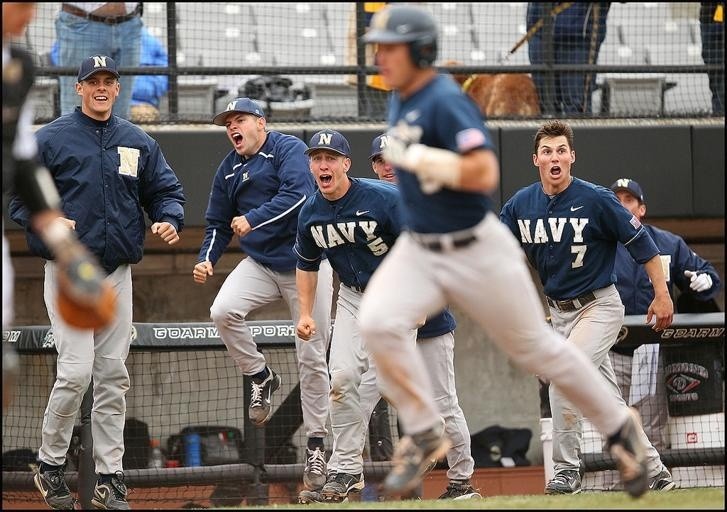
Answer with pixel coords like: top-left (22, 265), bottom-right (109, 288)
top-left (407, 234), bottom-right (476, 252)
top-left (62, 4), bottom-right (142, 25)
top-left (547, 291), bottom-right (596, 313)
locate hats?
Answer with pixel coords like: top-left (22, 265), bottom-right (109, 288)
top-left (609, 178), bottom-right (645, 201)
top-left (303, 129), bottom-right (390, 160)
top-left (77, 54), bottom-right (120, 83)
top-left (213, 98), bottom-right (265, 126)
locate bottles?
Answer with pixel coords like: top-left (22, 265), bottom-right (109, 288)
top-left (147, 429), bottom-right (202, 469)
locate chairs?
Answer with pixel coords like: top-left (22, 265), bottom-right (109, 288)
top-left (13, 2), bottom-right (714, 119)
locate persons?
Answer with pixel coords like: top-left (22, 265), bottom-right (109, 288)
top-left (54, 2), bottom-right (143, 126)
top-left (700, 2), bottom-right (725, 117)
top-left (292, 129), bottom-right (436, 502)
top-left (193, 97), bottom-right (333, 489)
top-left (8, 54), bottom-right (186, 509)
top-left (2, 1), bottom-right (42, 411)
top-left (526, 0), bottom-right (611, 118)
top-left (611, 178), bottom-right (721, 466)
top-left (500, 119), bottom-right (676, 495)
top-left (299, 127), bottom-right (482, 505)
top-left (51, 27), bottom-right (169, 123)
top-left (347, 1), bottom-right (395, 123)
top-left (359, 2), bottom-right (649, 498)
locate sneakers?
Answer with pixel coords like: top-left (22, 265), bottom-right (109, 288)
top-left (437, 484), bottom-right (482, 500)
top-left (298, 445), bottom-right (366, 505)
top-left (603, 415), bottom-right (676, 498)
top-left (544, 470), bottom-right (581, 495)
top-left (34, 463), bottom-right (74, 511)
top-left (384, 421), bottom-right (452, 495)
top-left (248, 367), bottom-right (282, 429)
top-left (91, 475), bottom-right (133, 510)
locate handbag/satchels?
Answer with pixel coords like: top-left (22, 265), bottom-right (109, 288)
top-left (168, 426), bottom-right (242, 465)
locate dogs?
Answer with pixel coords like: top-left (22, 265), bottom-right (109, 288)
top-left (441, 59), bottom-right (542, 116)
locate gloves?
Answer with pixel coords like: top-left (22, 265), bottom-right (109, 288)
top-left (381, 136), bottom-right (463, 196)
top-left (683, 270), bottom-right (713, 293)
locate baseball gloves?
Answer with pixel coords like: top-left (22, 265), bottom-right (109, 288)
top-left (58, 240), bottom-right (116, 329)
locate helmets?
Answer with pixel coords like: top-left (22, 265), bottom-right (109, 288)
top-left (360, 3), bottom-right (438, 68)
